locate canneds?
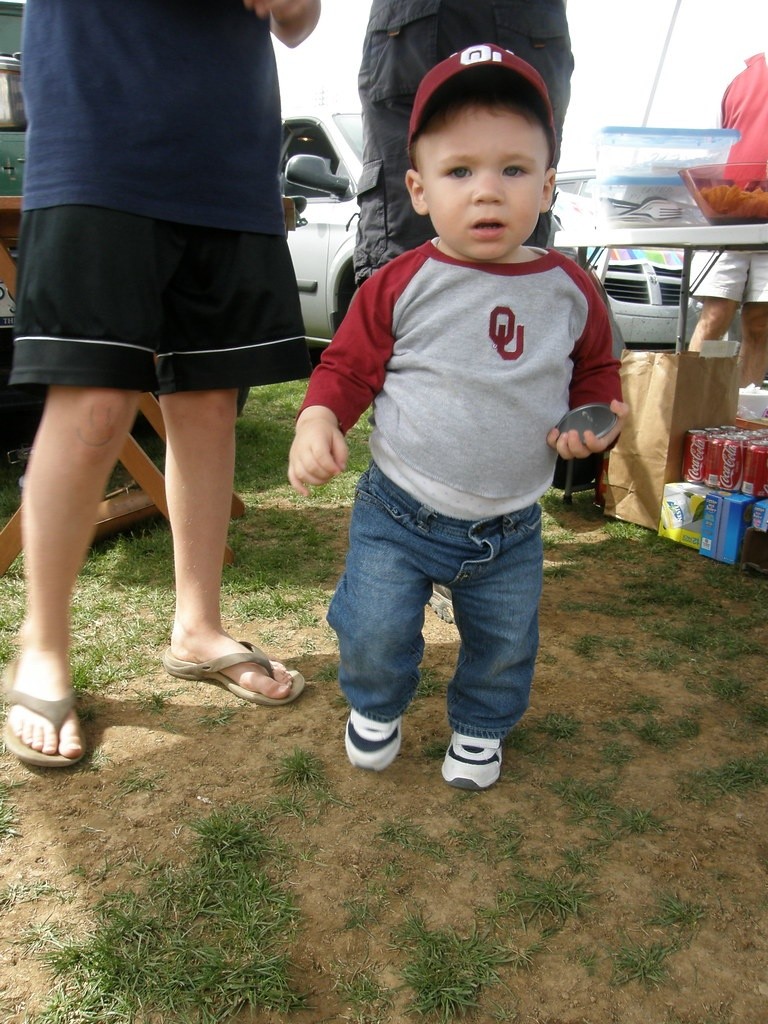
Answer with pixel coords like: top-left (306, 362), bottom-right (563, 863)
top-left (682, 425), bottom-right (768, 497)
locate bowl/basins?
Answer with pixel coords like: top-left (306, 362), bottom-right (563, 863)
top-left (679, 162), bottom-right (768, 224)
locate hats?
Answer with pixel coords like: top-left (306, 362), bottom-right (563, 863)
top-left (405, 42), bottom-right (557, 173)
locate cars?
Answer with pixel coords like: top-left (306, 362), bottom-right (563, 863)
top-left (278, 104), bottom-right (742, 350)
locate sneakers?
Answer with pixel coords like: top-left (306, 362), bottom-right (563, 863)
top-left (441, 728), bottom-right (503, 792)
top-left (343, 707), bottom-right (404, 773)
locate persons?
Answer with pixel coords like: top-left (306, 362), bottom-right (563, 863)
top-left (288, 44), bottom-right (628, 789)
top-left (2, 0), bottom-right (323, 766)
top-left (689, 52), bottom-right (768, 389)
top-left (345, 0), bottom-right (574, 621)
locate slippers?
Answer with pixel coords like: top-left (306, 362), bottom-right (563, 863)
top-left (0, 659), bottom-right (87, 768)
top-left (161, 639), bottom-right (305, 708)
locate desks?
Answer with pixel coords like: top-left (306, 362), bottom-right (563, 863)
top-left (553, 224), bottom-right (768, 504)
top-left (0, 196), bottom-right (296, 577)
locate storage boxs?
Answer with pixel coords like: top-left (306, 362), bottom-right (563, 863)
top-left (582, 126), bottom-right (741, 226)
top-left (657, 482), bottom-right (768, 573)
